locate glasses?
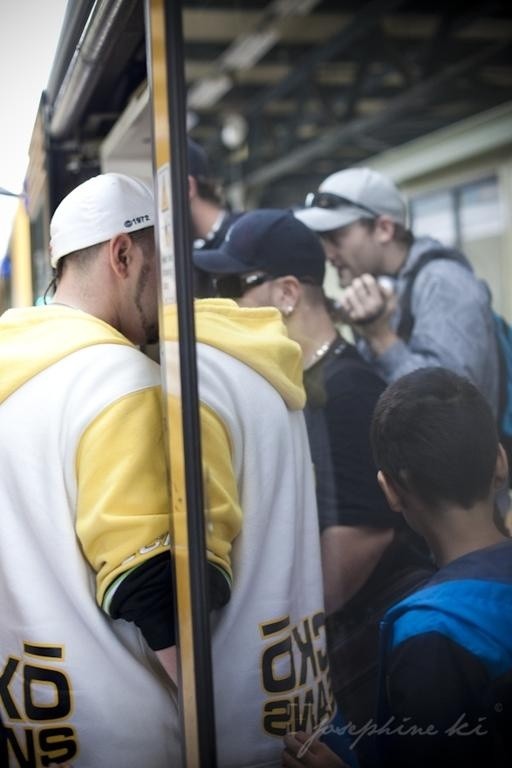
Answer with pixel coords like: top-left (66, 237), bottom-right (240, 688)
top-left (208, 270), bottom-right (280, 302)
top-left (304, 191), bottom-right (380, 219)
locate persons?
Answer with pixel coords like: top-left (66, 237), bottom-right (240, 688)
top-left (1, 168), bottom-right (187, 767)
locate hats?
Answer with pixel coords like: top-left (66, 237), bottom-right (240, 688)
top-left (49, 171), bottom-right (155, 269)
top-left (192, 208), bottom-right (325, 285)
top-left (186, 135), bottom-right (209, 177)
top-left (293, 167), bottom-right (409, 233)
